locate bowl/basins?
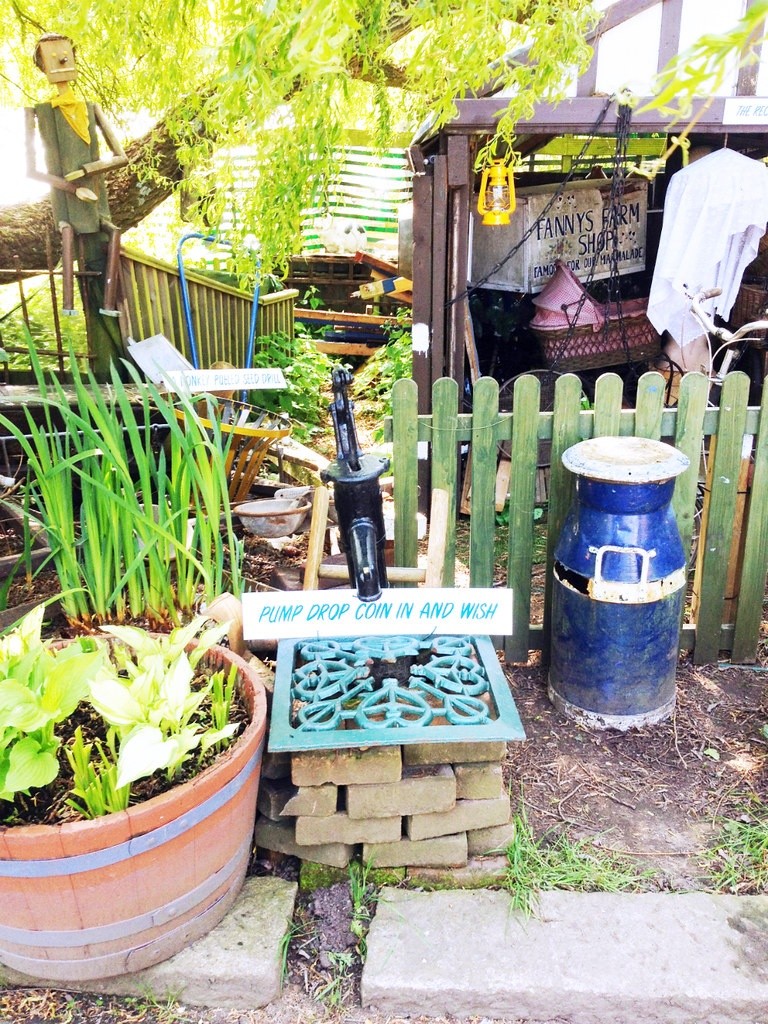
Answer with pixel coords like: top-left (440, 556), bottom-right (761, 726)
top-left (233, 497), bottom-right (311, 540)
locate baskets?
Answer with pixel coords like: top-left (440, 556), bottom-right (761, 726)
top-left (535, 314), bottom-right (666, 372)
top-left (732, 284), bottom-right (768, 349)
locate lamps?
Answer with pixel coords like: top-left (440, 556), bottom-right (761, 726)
top-left (476, 157), bottom-right (516, 225)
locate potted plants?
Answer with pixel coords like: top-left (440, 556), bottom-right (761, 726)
top-left (0, 320), bottom-right (274, 983)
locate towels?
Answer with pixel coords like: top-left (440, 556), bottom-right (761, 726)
top-left (641, 148), bottom-right (768, 348)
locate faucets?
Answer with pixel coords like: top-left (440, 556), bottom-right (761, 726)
top-left (319, 365), bottom-right (390, 602)
